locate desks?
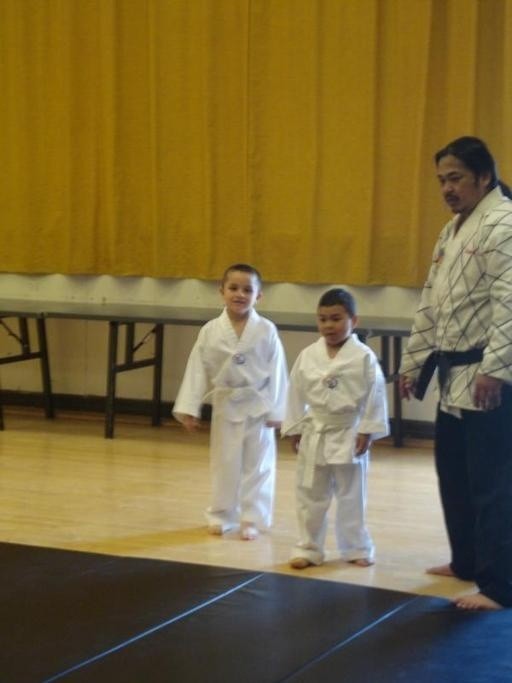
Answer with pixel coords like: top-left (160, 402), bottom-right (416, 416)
top-left (0, 295), bottom-right (430, 449)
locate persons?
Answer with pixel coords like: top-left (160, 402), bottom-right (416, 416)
top-left (171, 263), bottom-right (287, 541)
top-left (399, 134), bottom-right (509, 612)
top-left (280, 287), bottom-right (392, 572)
